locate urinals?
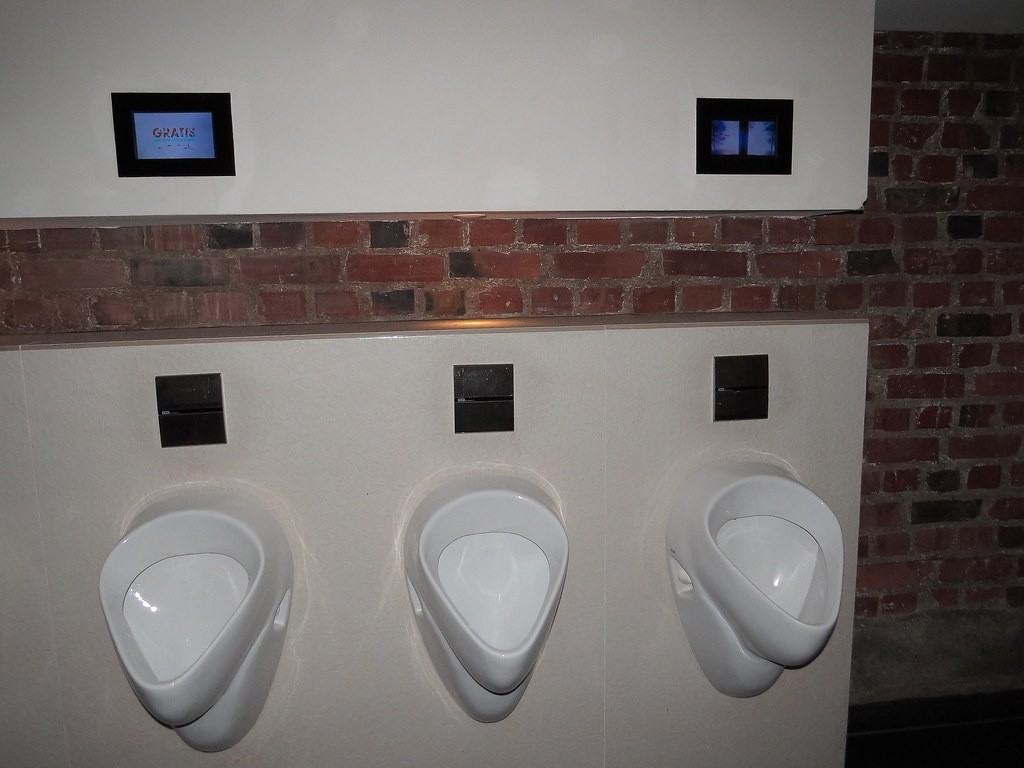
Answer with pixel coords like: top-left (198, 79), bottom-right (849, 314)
top-left (399, 475), bottom-right (570, 726)
top-left (664, 454), bottom-right (845, 699)
top-left (95, 490), bottom-right (294, 754)
